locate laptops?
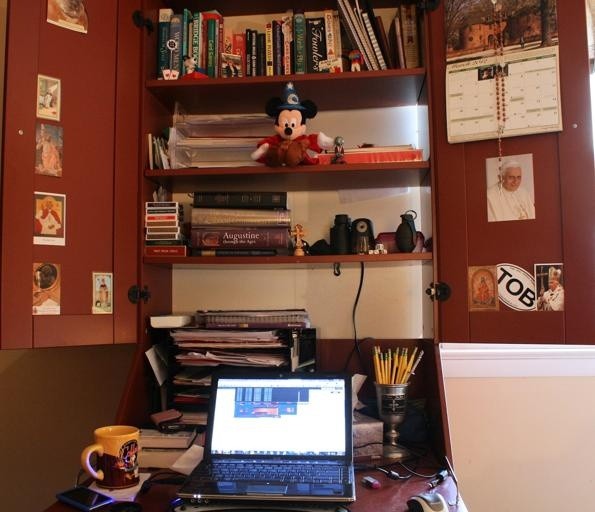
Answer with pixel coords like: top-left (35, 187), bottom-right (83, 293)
top-left (174, 371), bottom-right (357, 505)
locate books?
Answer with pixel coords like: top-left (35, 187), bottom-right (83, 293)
top-left (148, 133), bottom-right (172, 170)
top-left (188, 191), bottom-right (292, 257)
top-left (170, 308), bottom-right (311, 347)
top-left (140, 429), bottom-right (198, 450)
top-left (156, 0), bottom-right (422, 81)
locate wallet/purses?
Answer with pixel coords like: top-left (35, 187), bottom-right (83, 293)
top-left (151, 408), bottom-right (186, 434)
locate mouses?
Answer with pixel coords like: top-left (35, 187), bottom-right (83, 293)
top-left (406, 491), bottom-right (450, 512)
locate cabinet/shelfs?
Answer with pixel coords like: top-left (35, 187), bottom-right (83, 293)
top-left (0, 0), bottom-right (594, 351)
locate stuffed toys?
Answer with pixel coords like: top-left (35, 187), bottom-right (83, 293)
top-left (252, 83), bottom-right (336, 168)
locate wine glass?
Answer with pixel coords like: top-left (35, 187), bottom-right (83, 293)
top-left (375, 384), bottom-right (412, 459)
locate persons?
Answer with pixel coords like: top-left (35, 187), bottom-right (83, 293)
top-left (537, 267), bottom-right (564, 311)
top-left (35, 198), bottom-right (62, 234)
top-left (487, 161), bottom-right (535, 222)
top-left (100, 278), bottom-right (109, 306)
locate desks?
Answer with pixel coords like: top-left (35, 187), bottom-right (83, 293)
top-left (41, 336), bottom-right (470, 512)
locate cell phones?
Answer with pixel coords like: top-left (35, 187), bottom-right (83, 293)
top-left (54, 484), bottom-right (114, 512)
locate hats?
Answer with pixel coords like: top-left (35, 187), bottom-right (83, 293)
top-left (548, 267), bottom-right (561, 281)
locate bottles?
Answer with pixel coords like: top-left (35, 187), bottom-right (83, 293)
top-left (330, 213), bottom-right (352, 255)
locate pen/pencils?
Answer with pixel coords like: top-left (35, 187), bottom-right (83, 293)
top-left (373, 346), bottom-right (424, 386)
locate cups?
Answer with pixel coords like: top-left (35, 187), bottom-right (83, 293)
top-left (80, 423), bottom-right (140, 489)
